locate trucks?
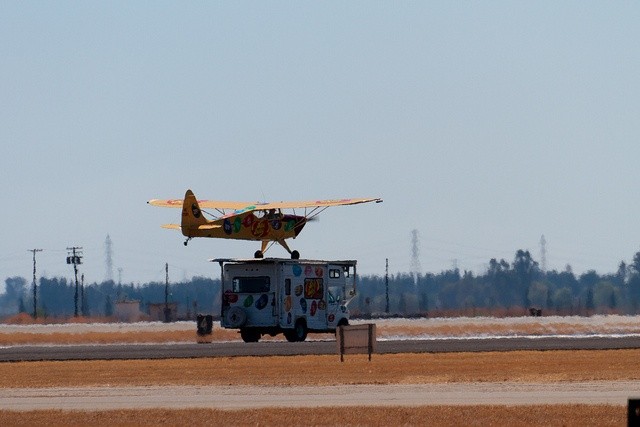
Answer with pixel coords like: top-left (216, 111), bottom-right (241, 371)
top-left (208, 257), bottom-right (358, 343)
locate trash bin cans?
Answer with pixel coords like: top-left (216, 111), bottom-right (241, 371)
top-left (197, 314), bottom-right (213, 343)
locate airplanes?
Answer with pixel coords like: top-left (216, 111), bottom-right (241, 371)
top-left (144, 187), bottom-right (385, 260)
